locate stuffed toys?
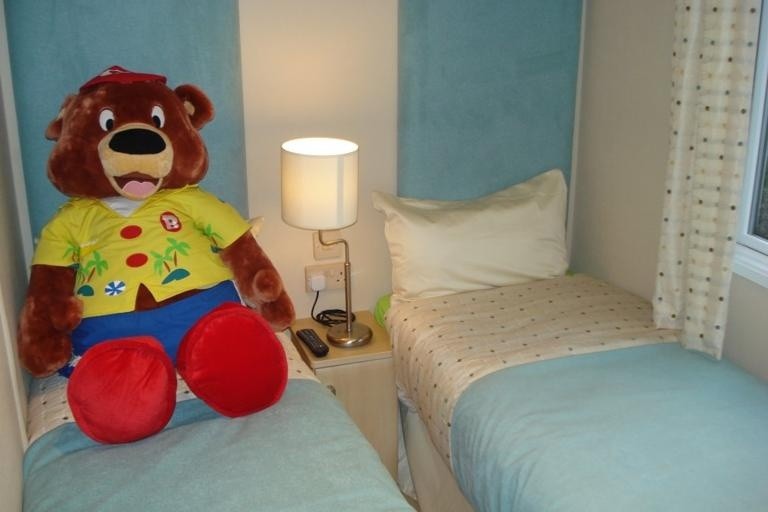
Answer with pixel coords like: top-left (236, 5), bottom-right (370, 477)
top-left (14, 64), bottom-right (297, 445)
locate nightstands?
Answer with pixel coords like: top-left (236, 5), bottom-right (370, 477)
top-left (286, 311), bottom-right (399, 488)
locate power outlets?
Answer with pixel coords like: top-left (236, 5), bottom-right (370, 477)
top-left (305, 261), bottom-right (346, 293)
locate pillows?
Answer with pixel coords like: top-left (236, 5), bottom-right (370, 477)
top-left (370, 164), bottom-right (571, 309)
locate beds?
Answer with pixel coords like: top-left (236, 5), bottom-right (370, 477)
top-left (23, 331), bottom-right (418, 512)
top-left (372, 268), bottom-right (767, 512)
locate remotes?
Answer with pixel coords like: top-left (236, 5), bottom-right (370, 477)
top-left (295, 327), bottom-right (330, 357)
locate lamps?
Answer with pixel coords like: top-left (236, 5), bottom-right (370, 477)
top-left (280, 136), bottom-right (374, 347)
